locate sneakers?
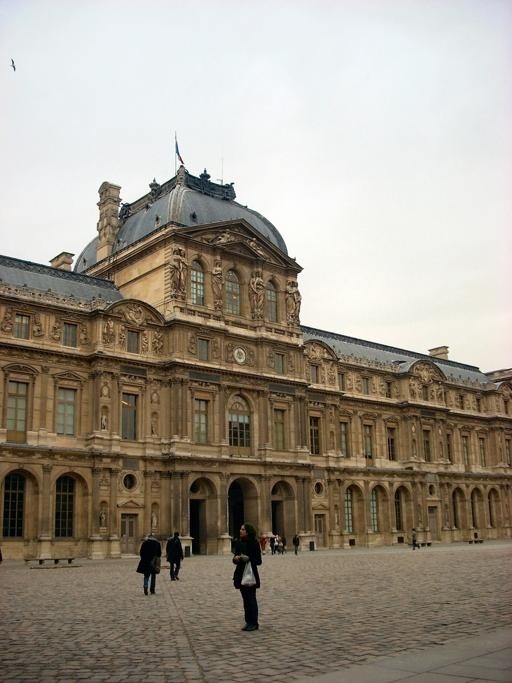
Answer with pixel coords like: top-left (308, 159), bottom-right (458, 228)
top-left (144, 591), bottom-right (155, 595)
top-left (171, 576), bottom-right (179, 581)
top-left (241, 623), bottom-right (259, 631)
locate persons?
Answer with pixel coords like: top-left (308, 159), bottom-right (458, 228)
top-left (249, 269), bottom-right (262, 321)
top-left (411, 528), bottom-right (420, 550)
top-left (231, 524), bottom-right (262, 631)
top-left (260, 534), bottom-right (267, 555)
top-left (136, 534), bottom-right (161, 595)
top-left (166, 532), bottom-right (183, 580)
top-left (170, 249), bottom-right (188, 300)
top-left (270, 534), bottom-right (286, 555)
top-left (287, 280), bottom-right (300, 323)
top-left (211, 256), bottom-right (225, 310)
top-left (293, 535), bottom-right (299, 555)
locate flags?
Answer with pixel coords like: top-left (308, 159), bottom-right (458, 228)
top-left (175, 139), bottom-right (183, 164)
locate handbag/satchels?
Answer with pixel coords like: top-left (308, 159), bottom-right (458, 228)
top-left (151, 556), bottom-right (161, 574)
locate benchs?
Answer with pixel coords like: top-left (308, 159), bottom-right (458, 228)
top-left (465, 539), bottom-right (483, 544)
top-left (25, 558), bottom-right (75, 564)
top-left (409, 541), bottom-right (432, 547)
